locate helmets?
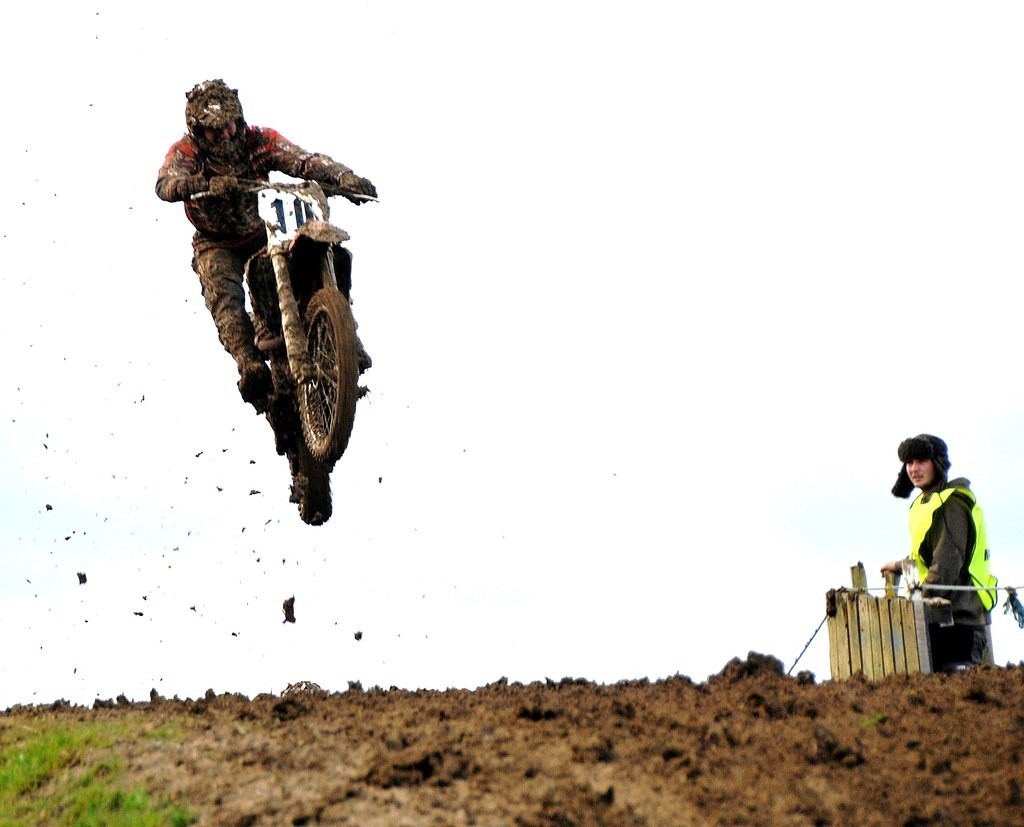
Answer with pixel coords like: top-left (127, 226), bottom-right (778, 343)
top-left (185, 80), bottom-right (245, 153)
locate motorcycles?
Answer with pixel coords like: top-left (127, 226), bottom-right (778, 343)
top-left (192, 173), bottom-right (379, 526)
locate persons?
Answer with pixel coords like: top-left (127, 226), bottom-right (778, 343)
top-left (880, 434), bottom-right (997, 676)
top-left (156, 79), bottom-right (378, 404)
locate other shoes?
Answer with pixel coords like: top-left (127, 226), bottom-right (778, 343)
top-left (241, 363), bottom-right (271, 402)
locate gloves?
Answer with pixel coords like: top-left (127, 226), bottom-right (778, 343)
top-left (209, 177), bottom-right (238, 196)
top-left (338, 171), bottom-right (378, 205)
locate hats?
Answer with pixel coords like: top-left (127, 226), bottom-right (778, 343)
top-left (891, 434), bottom-right (950, 499)
